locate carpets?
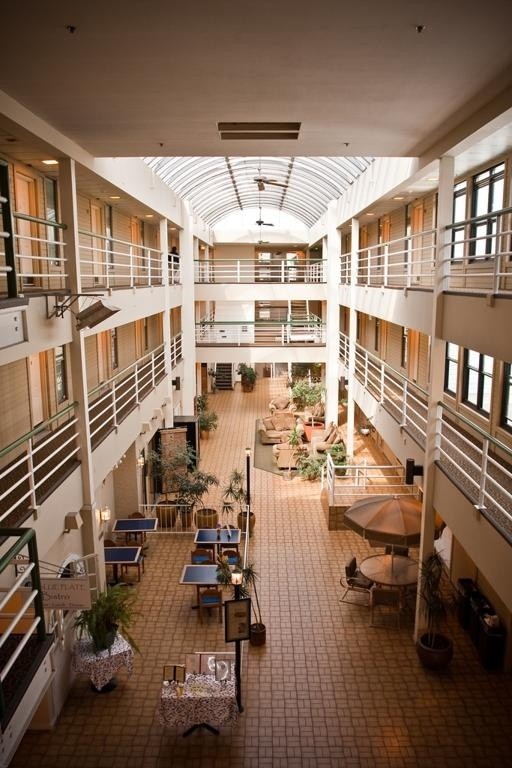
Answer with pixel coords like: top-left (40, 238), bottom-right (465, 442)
top-left (253, 435), bottom-right (328, 476)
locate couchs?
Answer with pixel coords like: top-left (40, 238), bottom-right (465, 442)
top-left (257, 398), bottom-right (347, 468)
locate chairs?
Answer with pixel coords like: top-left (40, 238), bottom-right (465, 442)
top-left (104, 512), bottom-right (146, 584)
top-left (339, 546), bottom-right (446, 631)
top-left (192, 525), bottom-right (241, 624)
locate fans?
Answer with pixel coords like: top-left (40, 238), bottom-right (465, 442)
top-left (253, 168), bottom-right (290, 245)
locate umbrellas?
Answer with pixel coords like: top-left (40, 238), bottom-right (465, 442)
top-left (343, 495), bottom-right (446, 575)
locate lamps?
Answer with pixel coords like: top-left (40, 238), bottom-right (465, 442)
top-left (63, 512), bottom-right (84, 533)
top-left (406, 458), bottom-right (423, 484)
top-left (361, 425), bottom-right (376, 437)
top-left (101, 507), bottom-right (111, 521)
top-left (46, 294), bottom-right (121, 331)
top-left (340, 377), bottom-right (348, 392)
top-left (172, 377), bottom-right (180, 390)
top-left (135, 455), bottom-right (144, 469)
top-left (140, 397), bottom-right (172, 435)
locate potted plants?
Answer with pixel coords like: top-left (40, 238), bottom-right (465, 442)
top-left (235, 364), bottom-right (259, 392)
top-left (70, 582), bottom-right (144, 658)
top-left (145, 438), bottom-right (256, 532)
top-left (216, 561), bottom-right (266, 645)
top-left (328, 441), bottom-right (345, 476)
top-left (408, 548), bottom-right (458, 672)
top-left (198, 411), bottom-right (219, 440)
top-left (282, 428), bottom-right (304, 480)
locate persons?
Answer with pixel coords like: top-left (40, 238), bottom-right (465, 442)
top-left (168, 246), bottom-right (180, 273)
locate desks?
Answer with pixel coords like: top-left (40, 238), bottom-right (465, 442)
top-left (71, 630), bottom-right (134, 693)
top-left (156, 664), bottom-right (243, 737)
top-left (111, 518), bottom-right (159, 557)
top-left (179, 565), bottom-right (236, 608)
